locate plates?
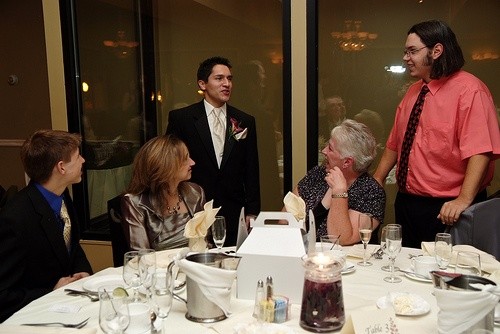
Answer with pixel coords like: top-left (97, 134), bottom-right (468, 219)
top-left (376, 293), bottom-right (431, 316)
top-left (404, 267), bottom-right (455, 282)
top-left (235, 323), bottom-right (297, 334)
top-left (316, 242), bottom-right (342, 252)
top-left (340, 262), bottom-right (356, 274)
top-left (83, 275), bottom-right (131, 291)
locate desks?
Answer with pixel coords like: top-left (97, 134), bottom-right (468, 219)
top-left (0, 240), bottom-right (498, 334)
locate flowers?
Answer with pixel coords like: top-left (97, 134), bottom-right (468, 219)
top-left (227, 117), bottom-right (242, 141)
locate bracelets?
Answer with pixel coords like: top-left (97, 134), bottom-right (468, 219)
top-left (248, 214), bottom-right (258, 220)
top-left (331, 192), bottom-right (350, 199)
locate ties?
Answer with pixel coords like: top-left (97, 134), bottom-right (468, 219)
top-left (212, 107), bottom-right (226, 166)
top-left (397, 84), bottom-right (430, 193)
top-left (59, 200), bottom-right (72, 254)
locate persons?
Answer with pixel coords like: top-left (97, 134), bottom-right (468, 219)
top-left (83, 78), bottom-right (144, 168)
top-left (121, 135), bottom-right (208, 261)
top-left (227, 58), bottom-right (386, 175)
top-left (162, 56), bottom-right (261, 247)
top-left (0, 129), bottom-right (94, 323)
top-left (277, 118), bottom-right (387, 246)
top-left (373, 19), bottom-right (500, 250)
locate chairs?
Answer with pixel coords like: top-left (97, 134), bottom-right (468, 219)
top-left (454, 195), bottom-right (500, 260)
top-left (111, 193), bottom-right (144, 261)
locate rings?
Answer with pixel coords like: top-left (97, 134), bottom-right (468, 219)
top-left (327, 172), bottom-right (330, 175)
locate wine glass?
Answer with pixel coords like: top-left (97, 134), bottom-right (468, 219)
top-left (212, 217), bottom-right (226, 252)
top-left (357, 213), bottom-right (373, 266)
top-left (122, 249), bottom-right (172, 334)
top-left (380, 224), bottom-right (402, 283)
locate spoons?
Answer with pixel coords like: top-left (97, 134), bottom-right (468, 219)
top-left (381, 266), bottom-right (428, 278)
top-left (64, 288), bottom-right (99, 302)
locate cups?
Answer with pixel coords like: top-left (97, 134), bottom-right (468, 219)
top-left (260, 302), bottom-right (292, 323)
top-left (320, 235), bottom-right (340, 253)
top-left (99, 285), bottom-right (131, 334)
top-left (410, 256), bottom-right (437, 279)
top-left (456, 251), bottom-right (481, 276)
top-left (434, 233), bottom-right (453, 270)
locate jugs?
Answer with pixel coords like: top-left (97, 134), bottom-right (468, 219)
top-left (429, 270), bottom-right (498, 334)
top-left (166, 252), bottom-right (242, 323)
top-left (298, 252), bottom-right (346, 334)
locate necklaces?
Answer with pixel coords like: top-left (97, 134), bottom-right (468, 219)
top-left (165, 191), bottom-right (182, 214)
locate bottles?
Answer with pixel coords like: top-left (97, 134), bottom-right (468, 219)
top-left (264, 275), bottom-right (274, 301)
top-left (253, 280), bottom-right (265, 318)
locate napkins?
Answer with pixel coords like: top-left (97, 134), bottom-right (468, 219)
top-left (186, 198), bottom-right (220, 253)
top-left (284, 193), bottom-right (307, 225)
top-left (420, 241), bottom-right (495, 271)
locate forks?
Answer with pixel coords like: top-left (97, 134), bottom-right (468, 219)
top-left (21, 316), bottom-right (91, 329)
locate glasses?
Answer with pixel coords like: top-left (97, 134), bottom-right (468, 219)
top-left (403, 43), bottom-right (435, 57)
